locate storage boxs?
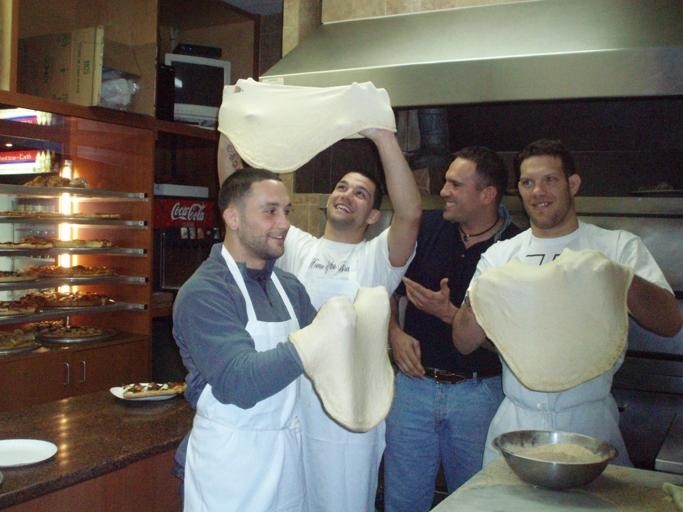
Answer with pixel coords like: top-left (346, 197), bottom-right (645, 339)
top-left (17, 26), bottom-right (141, 105)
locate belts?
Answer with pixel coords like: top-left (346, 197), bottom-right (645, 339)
top-left (424, 365), bottom-right (499, 385)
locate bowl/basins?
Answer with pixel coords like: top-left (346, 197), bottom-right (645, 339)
top-left (493, 429), bottom-right (620, 491)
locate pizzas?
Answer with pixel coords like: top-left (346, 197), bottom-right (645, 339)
top-left (33, 264), bottom-right (105, 276)
top-left (1, 270), bottom-right (30, 282)
top-left (122, 383), bottom-right (186, 400)
top-left (42, 326), bottom-right (101, 339)
top-left (24, 321), bottom-right (63, 334)
top-left (0, 301), bottom-right (36, 316)
top-left (28, 291), bottom-right (108, 309)
top-left (71, 213), bottom-right (121, 219)
top-left (54, 238), bottom-right (114, 249)
top-left (2, 212), bottom-right (71, 219)
top-left (0, 237), bottom-right (53, 250)
top-left (0, 331), bottom-right (32, 353)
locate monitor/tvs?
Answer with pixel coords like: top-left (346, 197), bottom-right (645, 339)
top-left (165, 53), bottom-right (232, 122)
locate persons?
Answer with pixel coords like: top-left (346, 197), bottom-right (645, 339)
top-left (171, 170), bottom-right (391, 508)
top-left (454, 137), bottom-right (682, 470)
top-left (387, 148), bottom-right (524, 507)
top-left (218, 76), bottom-right (424, 507)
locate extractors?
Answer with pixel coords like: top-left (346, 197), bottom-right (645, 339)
top-left (256, 1), bottom-right (683, 219)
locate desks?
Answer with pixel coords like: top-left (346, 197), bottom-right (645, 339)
top-left (428, 456), bottom-right (683, 511)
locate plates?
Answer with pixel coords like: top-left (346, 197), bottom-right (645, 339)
top-left (108, 381), bottom-right (179, 402)
top-left (37, 329), bottom-right (113, 345)
top-left (1, 437), bottom-right (57, 468)
top-left (0, 341), bottom-right (41, 356)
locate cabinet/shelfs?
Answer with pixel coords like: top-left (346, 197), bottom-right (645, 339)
top-left (0, 341), bottom-right (147, 408)
top-left (0, 0), bottom-right (260, 404)
